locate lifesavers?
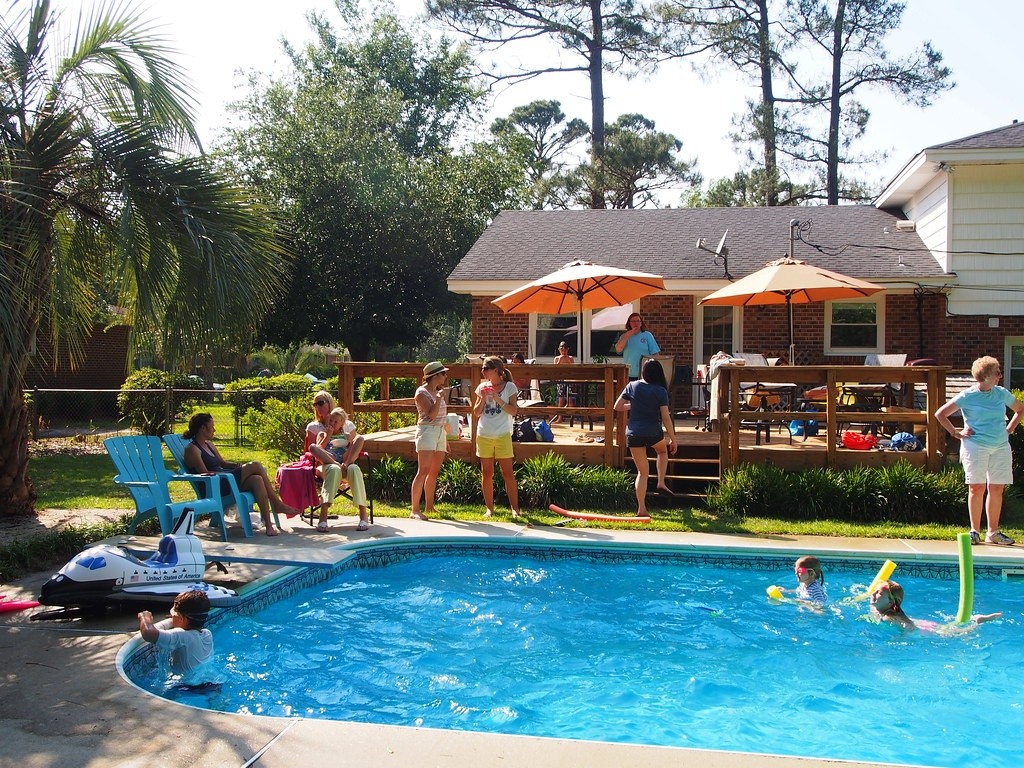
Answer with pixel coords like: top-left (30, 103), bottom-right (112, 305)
top-left (956, 532), bottom-right (974, 622)
top-left (766, 560), bottom-right (896, 598)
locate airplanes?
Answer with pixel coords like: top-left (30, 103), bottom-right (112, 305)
top-left (37, 507), bottom-right (236, 620)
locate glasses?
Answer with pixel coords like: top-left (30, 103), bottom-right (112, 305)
top-left (169, 608), bottom-right (182, 618)
top-left (435, 372), bottom-right (446, 376)
top-left (991, 370), bottom-right (1001, 377)
top-left (480, 366), bottom-right (494, 371)
top-left (628, 319), bottom-right (641, 324)
top-left (794, 567), bottom-right (814, 574)
top-left (311, 400), bottom-right (326, 407)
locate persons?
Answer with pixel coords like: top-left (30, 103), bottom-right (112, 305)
top-left (935, 356), bottom-right (1024, 545)
top-left (779, 555), bottom-right (828, 604)
top-left (473, 356), bottom-right (520, 518)
top-left (554, 340), bottom-right (574, 425)
top-left (618, 313), bottom-right (661, 382)
top-left (138, 590), bottom-right (214, 669)
top-left (306, 391), bottom-right (369, 531)
top-left (182, 413), bottom-right (299, 535)
top-left (409, 361), bottom-right (449, 520)
top-left (510, 352), bottom-right (525, 396)
top-left (309, 407), bottom-right (365, 471)
top-left (613, 358), bottom-right (678, 517)
top-left (870, 581), bottom-right (1002, 624)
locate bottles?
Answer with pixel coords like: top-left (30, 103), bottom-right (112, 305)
top-left (487, 381), bottom-right (494, 398)
top-left (696, 370), bottom-right (701, 383)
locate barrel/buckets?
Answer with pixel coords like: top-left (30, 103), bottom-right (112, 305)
top-left (446, 415), bottom-right (459, 440)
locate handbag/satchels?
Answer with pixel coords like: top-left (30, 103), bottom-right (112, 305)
top-left (788, 410), bottom-right (820, 435)
top-left (890, 431), bottom-right (924, 452)
top-left (512, 419), bottom-right (556, 443)
top-left (841, 431), bottom-right (879, 451)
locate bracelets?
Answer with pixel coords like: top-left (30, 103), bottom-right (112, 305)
top-left (501, 403), bottom-right (506, 408)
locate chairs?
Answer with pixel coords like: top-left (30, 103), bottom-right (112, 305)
top-left (103, 435), bottom-right (229, 540)
top-left (839, 353), bottom-right (908, 439)
top-left (164, 434), bottom-right (281, 538)
top-left (636, 353), bottom-right (678, 431)
top-left (302, 436), bottom-right (374, 526)
top-left (447, 353), bottom-right (547, 424)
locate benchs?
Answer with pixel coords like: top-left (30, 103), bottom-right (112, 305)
top-left (881, 373), bottom-right (980, 416)
top-left (808, 381), bottom-right (883, 406)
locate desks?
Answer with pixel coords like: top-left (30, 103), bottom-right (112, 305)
top-left (553, 380), bottom-right (618, 431)
top-left (796, 398), bottom-right (841, 439)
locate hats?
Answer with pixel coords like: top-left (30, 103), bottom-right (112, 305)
top-left (422, 361), bottom-right (449, 379)
top-left (557, 341), bottom-right (569, 349)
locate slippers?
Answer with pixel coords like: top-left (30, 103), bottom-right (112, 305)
top-left (355, 521), bottom-right (370, 530)
top-left (317, 521), bottom-right (328, 533)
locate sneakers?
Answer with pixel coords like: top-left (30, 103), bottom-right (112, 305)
top-left (969, 531), bottom-right (979, 545)
top-left (986, 532), bottom-right (1016, 545)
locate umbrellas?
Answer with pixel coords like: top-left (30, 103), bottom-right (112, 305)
top-left (698, 252), bottom-right (887, 345)
top-left (492, 257), bottom-right (666, 364)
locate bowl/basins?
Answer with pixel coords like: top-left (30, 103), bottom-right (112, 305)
top-left (330, 439), bottom-right (348, 447)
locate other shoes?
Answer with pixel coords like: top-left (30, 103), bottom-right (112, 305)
top-left (266, 525), bottom-right (278, 536)
